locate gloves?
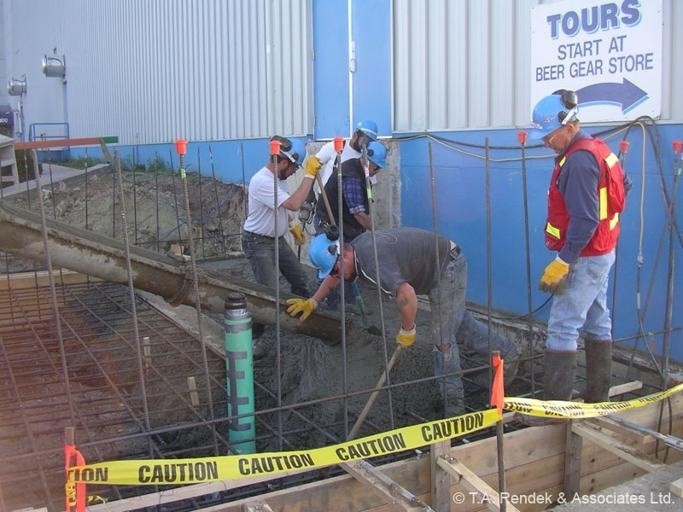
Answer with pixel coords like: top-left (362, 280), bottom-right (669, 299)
top-left (395, 324), bottom-right (417, 348)
top-left (304, 155), bottom-right (322, 179)
top-left (539, 253), bottom-right (571, 295)
top-left (286, 296), bottom-right (317, 323)
top-left (290, 224), bottom-right (305, 246)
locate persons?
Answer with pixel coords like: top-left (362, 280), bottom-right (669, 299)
top-left (313, 141), bottom-right (387, 316)
top-left (300, 118), bottom-right (378, 203)
top-left (285, 228), bottom-right (520, 421)
top-left (241, 135), bottom-right (323, 359)
top-left (512, 90), bottom-right (633, 426)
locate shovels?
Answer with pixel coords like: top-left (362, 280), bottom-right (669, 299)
top-left (316, 171), bottom-right (383, 336)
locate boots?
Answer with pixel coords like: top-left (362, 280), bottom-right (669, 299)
top-left (542, 349), bottom-right (577, 402)
top-left (584, 339), bottom-right (612, 403)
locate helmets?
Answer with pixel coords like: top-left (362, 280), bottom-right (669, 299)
top-left (361, 140), bottom-right (387, 169)
top-left (530, 88), bottom-right (579, 141)
top-left (355, 119), bottom-right (378, 142)
top-left (308, 232), bottom-right (340, 280)
top-left (269, 135), bottom-right (307, 170)
